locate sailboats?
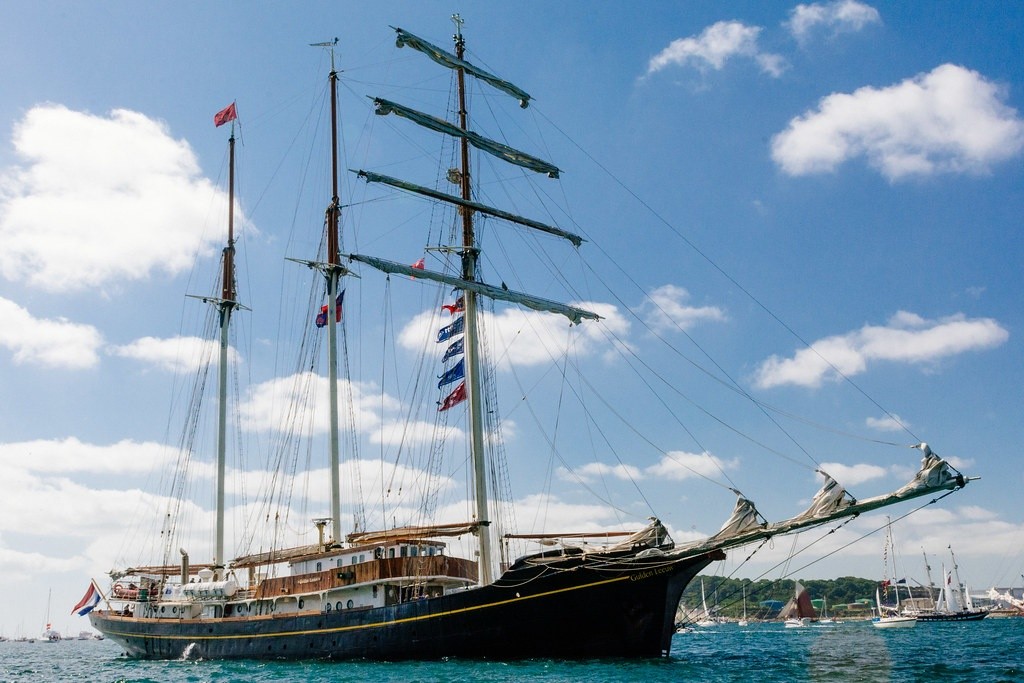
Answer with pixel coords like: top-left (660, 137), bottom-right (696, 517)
top-left (0, 587), bottom-right (105, 644)
top-left (674, 515), bottom-right (1024, 635)
top-left (87, 11), bottom-right (982, 664)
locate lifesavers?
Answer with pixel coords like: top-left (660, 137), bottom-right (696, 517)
top-left (114, 584), bottom-right (124, 595)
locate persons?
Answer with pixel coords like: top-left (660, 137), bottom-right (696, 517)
top-left (124, 604), bottom-right (129, 616)
top-left (190, 577), bottom-right (202, 583)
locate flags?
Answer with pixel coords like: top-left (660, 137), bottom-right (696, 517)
top-left (69, 583), bottom-right (101, 617)
top-left (440, 335), bottom-right (464, 362)
top-left (439, 295), bottom-right (465, 317)
top-left (213, 102), bottom-right (237, 127)
top-left (316, 288), bottom-right (346, 328)
top-left (435, 312), bottom-right (466, 343)
top-left (410, 257), bottom-right (425, 279)
top-left (439, 379), bottom-right (468, 411)
top-left (437, 355), bottom-right (464, 388)
